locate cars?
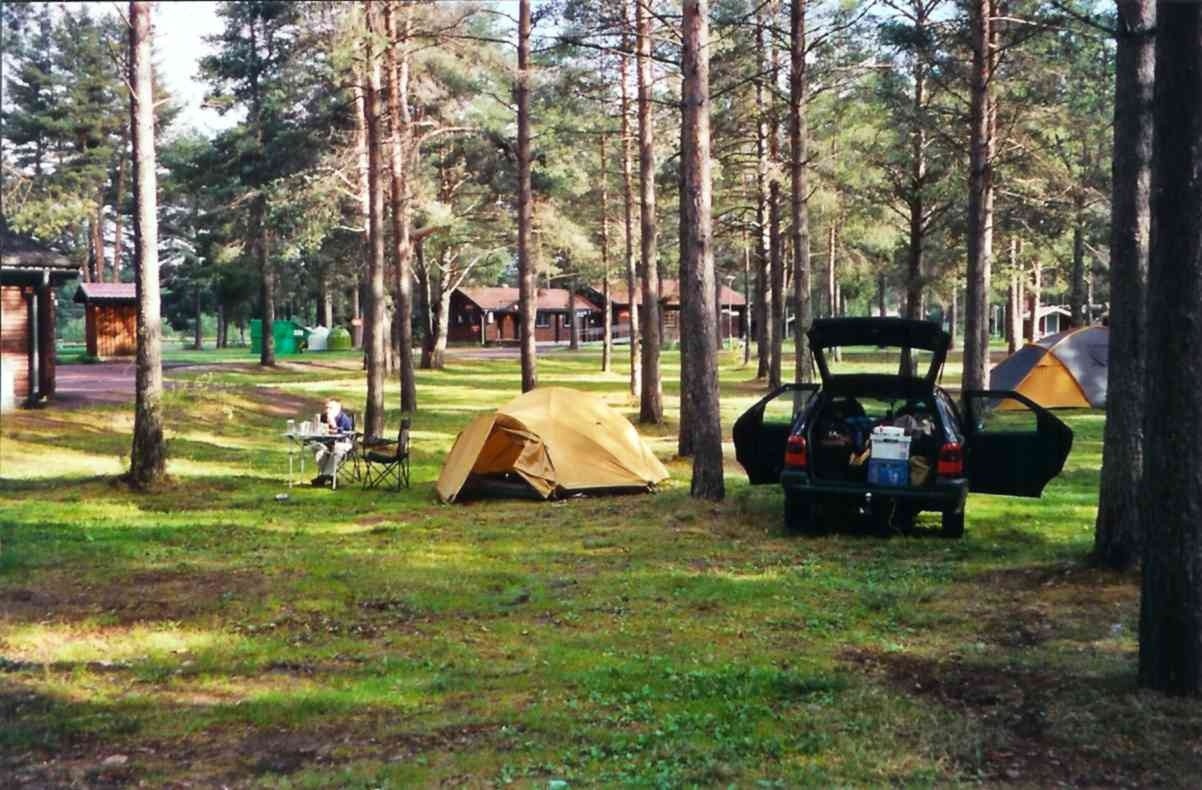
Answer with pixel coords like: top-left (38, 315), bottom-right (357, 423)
top-left (730, 315), bottom-right (1076, 540)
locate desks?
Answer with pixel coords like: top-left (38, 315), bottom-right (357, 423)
top-left (282, 431), bottom-right (348, 490)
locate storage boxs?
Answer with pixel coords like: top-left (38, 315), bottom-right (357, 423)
top-left (909, 455), bottom-right (934, 486)
top-left (867, 459), bottom-right (911, 489)
top-left (870, 434), bottom-right (912, 460)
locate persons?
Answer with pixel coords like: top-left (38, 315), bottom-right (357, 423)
top-left (308, 397), bottom-right (354, 489)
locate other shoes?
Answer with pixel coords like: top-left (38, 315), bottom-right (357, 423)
top-left (312, 475), bottom-right (333, 486)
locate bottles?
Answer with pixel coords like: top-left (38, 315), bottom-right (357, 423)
top-left (274, 494), bottom-right (288, 500)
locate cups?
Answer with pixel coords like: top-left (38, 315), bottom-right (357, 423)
top-left (313, 414), bottom-right (320, 432)
top-left (297, 423), bottom-right (305, 432)
top-left (304, 421), bottom-right (313, 432)
top-left (321, 423), bottom-right (329, 433)
top-left (287, 419), bottom-right (295, 433)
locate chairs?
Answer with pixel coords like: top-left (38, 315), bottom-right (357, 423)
top-left (361, 417), bottom-right (411, 492)
top-left (310, 410), bottom-right (361, 484)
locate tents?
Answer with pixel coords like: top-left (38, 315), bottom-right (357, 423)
top-left (986, 322), bottom-right (1109, 416)
top-left (433, 387), bottom-right (671, 508)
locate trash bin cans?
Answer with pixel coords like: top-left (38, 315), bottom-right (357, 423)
top-left (249, 318), bottom-right (311, 354)
